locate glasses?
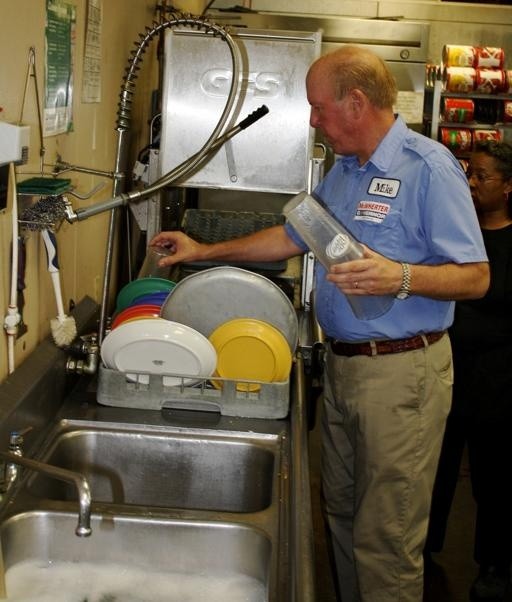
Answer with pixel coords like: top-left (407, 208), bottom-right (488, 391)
top-left (466, 170), bottom-right (504, 183)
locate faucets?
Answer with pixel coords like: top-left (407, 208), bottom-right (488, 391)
top-left (0, 449), bottom-right (93, 537)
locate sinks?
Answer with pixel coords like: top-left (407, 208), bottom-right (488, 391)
top-left (25, 430), bottom-right (276, 515)
top-left (0, 510), bottom-right (272, 602)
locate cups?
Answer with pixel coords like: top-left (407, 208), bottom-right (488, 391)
top-left (282, 190), bottom-right (395, 321)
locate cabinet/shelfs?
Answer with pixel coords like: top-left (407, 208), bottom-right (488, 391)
top-left (421, 80), bottom-right (512, 177)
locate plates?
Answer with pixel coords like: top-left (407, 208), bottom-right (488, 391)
top-left (100, 276), bottom-right (293, 391)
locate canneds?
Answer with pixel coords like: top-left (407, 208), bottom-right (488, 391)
top-left (425, 43), bottom-right (512, 174)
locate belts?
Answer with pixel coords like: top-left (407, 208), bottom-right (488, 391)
top-left (327, 327), bottom-right (446, 360)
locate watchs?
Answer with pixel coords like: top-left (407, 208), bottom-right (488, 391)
top-left (394, 263), bottom-right (410, 299)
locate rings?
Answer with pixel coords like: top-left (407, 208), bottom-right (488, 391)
top-left (354, 281), bottom-right (358, 288)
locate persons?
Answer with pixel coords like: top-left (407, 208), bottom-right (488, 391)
top-left (148, 42), bottom-right (489, 601)
top-left (422, 139), bottom-right (512, 601)
